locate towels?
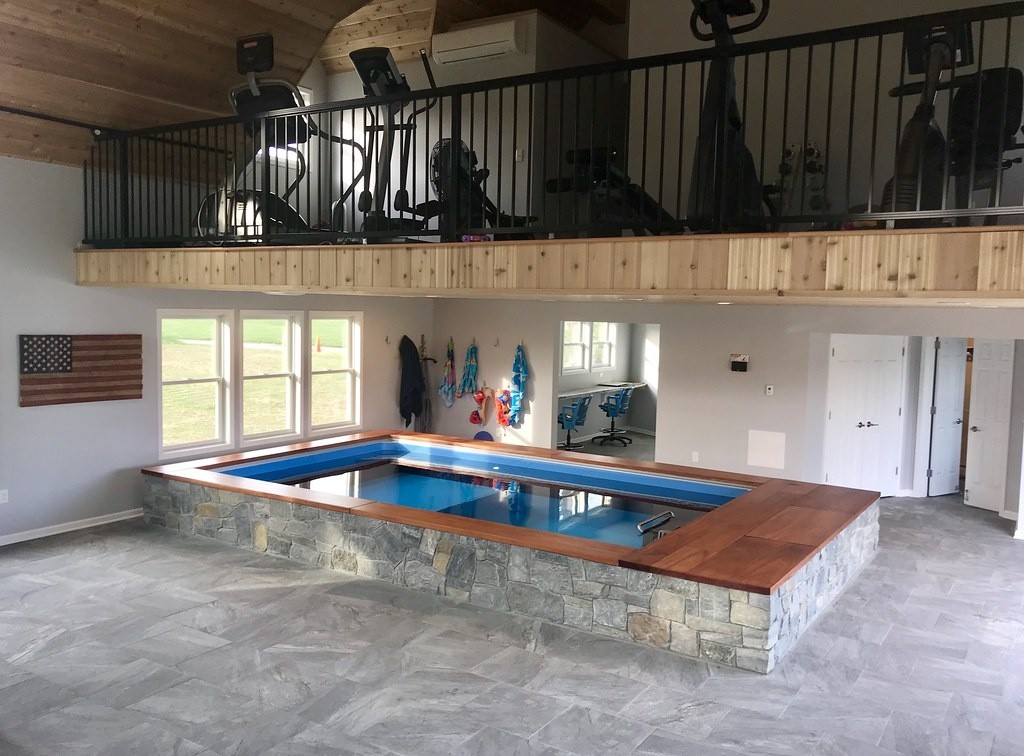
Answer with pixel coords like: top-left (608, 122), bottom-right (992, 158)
top-left (456, 344), bottom-right (479, 399)
top-left (437, 340), bottom-right (457, 408)
top-left (507, 344), bottom-right (529, 426)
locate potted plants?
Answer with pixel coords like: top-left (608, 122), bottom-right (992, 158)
top-left (431, 18), bottom-right (532, 66)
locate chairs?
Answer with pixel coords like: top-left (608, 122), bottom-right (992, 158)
top-left (592, 387), bottom-right (634, 447)
top-left (558, 394), bottom-right (593, 451)
top-left (940, 65), bottom-right (1023, 226)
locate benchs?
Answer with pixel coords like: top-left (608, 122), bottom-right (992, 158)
top-left (565, 146), bottom-right (684, 234)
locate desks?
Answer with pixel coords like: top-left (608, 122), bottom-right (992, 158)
top-left (558, 381), bottom-right (647, 401)
